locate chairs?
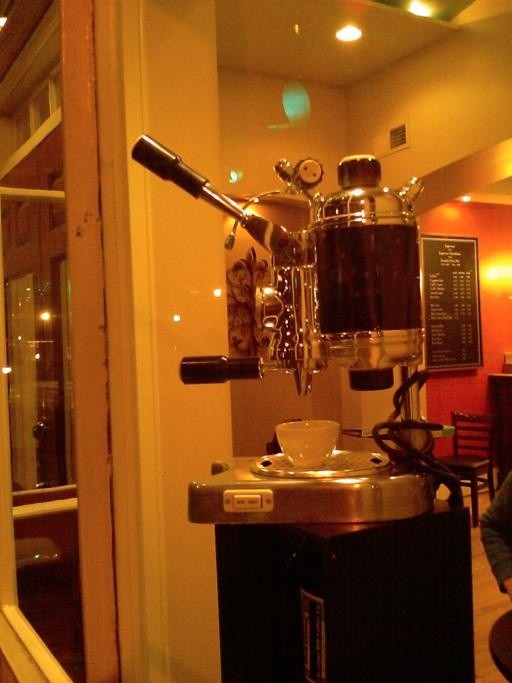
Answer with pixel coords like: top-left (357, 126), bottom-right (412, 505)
top-left (433, 411), bottom-right (502, 527)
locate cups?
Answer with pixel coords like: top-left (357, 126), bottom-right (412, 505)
top-left (273, 417), bottom-right (343, 474)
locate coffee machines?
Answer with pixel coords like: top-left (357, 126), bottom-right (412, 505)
top-left (129, 132), bottom-right (429, 397)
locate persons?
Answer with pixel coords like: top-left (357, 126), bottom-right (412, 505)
top-left (475, 471), bottom-right (511, 605)
